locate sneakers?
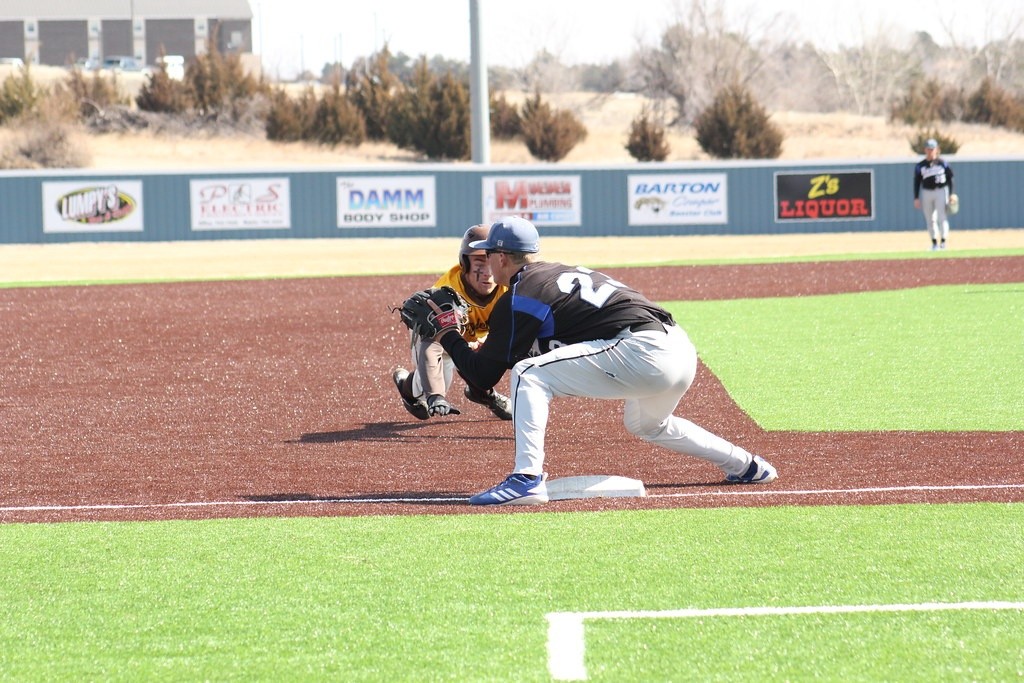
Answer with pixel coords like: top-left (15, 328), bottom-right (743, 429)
top-left (468, 472), bottom-right (550, 506)
top-left (464, 385), bottom-right (514, 420)
top-left (393, 368), bottom-right (430, 420)
top-left (720, 456), bottom-right (779, 483)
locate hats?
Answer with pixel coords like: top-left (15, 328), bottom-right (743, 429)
top-left (468, 215), bottom-right (539, 253)
top-left (925, 138), bottom-right (938, 148)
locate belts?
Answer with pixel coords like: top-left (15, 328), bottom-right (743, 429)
top-left (628, 322), bottom-right (667, 333)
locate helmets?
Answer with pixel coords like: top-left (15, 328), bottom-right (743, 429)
top-left (459, 223), bottom-right (492, 276)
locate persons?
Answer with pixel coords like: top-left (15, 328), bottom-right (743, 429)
top-left (397, 212), bottom-right (780, 505)
top-left (912, 136), bottom-right (958, 250)
top-left (389, 223), bottom-right (518, 422)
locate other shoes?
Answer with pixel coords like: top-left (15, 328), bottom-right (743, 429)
top-left (932, 244), bottom-right (937, 249)
top-left (940, 243), bottom-right (946, 248)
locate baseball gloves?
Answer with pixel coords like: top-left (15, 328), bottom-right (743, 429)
top-left (949, 194), bottom-right (958, 214)
top-left (385, 285), bottom-right (463, 350)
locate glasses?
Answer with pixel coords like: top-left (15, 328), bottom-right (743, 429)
top-left (485, 249), bottom-right (513, 261)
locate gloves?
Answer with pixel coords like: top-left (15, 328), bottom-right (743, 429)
top-left (426, 395), bottom-right (462, 417)
